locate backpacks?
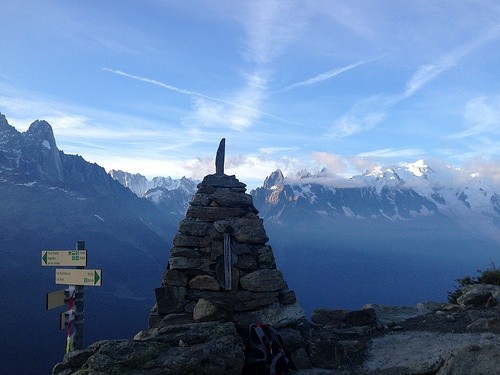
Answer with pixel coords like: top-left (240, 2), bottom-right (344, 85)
top-left (248, 323), bottom-right (299, 375)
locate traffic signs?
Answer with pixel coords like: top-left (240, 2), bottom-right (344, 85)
top-left (40, 246), bottom-right (102, 353)
